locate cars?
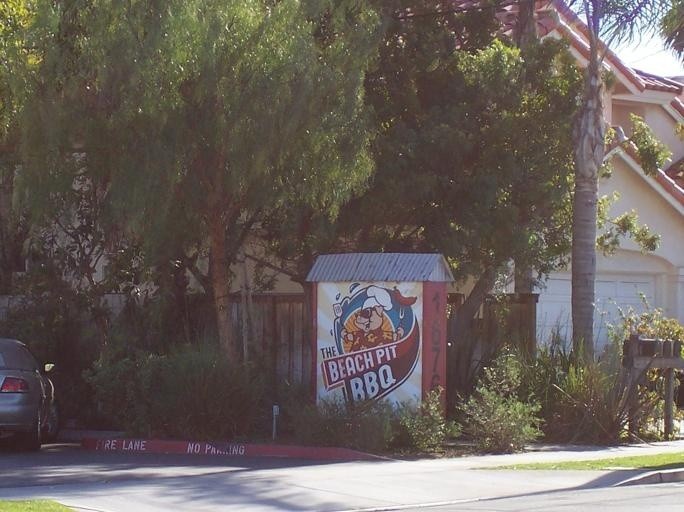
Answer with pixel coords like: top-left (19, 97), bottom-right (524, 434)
top-left (0, 338), bottom-right (61, 451)
